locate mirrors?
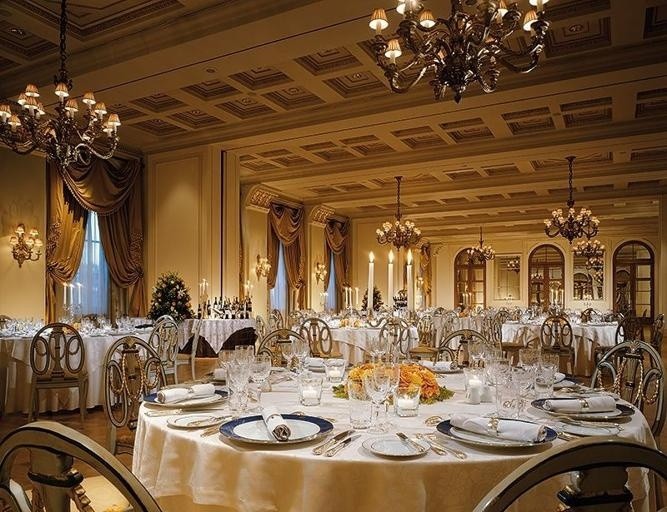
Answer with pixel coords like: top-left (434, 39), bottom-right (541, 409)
top-left (573, 247), bottom-right (606, 300)
top-left (493, 253), bottom-right (521, 301)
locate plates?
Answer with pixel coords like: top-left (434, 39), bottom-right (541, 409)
top-left (363, 434), bottom-right (432, 460)
top-left (217, 414), bottom-right (334, 445)
top-left (536, 369), bottom-right (566, 384)
top-left (168, 413), bottom-right (225, 428)
top-left (142, 385), bottom-right (222, 408)
top-left (437, 416), bottom-right (560, 446)
top-left (532, 395), bottom-right (636, 437)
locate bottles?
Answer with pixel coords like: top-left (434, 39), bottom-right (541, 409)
top-left (197, 297), bottom-right (251, 318)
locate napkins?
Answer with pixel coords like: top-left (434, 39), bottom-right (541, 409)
top-left (212, 368), bottom-right (226, 381)
top-left (156, 382), bottom-right (216, 405)
top-left (448, 413), bottom-right (548, 443)
top-left (541, 395), bottom-right (617, 414)
top-left (417, 359), bottom-right (459, 371)
top-left (261, 406), bottom-right (292, 442)
top-left (304, 357), bottom-right (325, 367)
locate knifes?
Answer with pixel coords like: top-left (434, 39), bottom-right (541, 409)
top-left (568, 385), bottom-right (610, 396)
top-left (396, 432), bottom-right (424, 451)
top-left (418, 429), bottom-right (466, 462)
top-left (313, 428), bottom-right (361, 456)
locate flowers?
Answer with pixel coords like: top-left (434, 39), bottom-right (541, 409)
top-left (142, 269), bottom-right (191, 319)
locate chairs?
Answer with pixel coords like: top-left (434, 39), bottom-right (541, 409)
top-left (100, 333), bottom-right (169, 458)
top-left (590, 338), bottom-right (666, 450)
top-left (2, 312), bottom-right (108, 330)
top-left (473, 435), bottom-right (666, 512)
top-left (252, 303), bottom-right (665, 381)
top-left (29, 321), bottom-right (91, 424)
top-left (145, 313), bottom-right (179, 388)
top-left (2, 420), bottom-right (163, 512)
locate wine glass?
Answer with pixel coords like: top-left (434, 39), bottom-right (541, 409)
top-left (217, 329), bottom-right (558, 427)
top-left (1, 316), bottom-right (148, 334)
top-left (306, 311), bottom-right (413, 326)
top-left (425, 303), bottom-right (617, 323)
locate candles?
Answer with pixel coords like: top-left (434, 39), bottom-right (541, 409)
top-left (368, 251), bottom-right (374, 309)
top-left (407, 250), bottom-right (413, 308)
top-left (388, 250), bottom-right (394, 309)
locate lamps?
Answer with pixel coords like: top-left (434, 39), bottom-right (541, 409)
top-left (363, 1), bottom-right (551, 104)
top-left (461, 221), bottom-right (498, 260)
top-left (253, 252), bottom-right (271, 280)
top-left (372, 174), bottom-right (422, 247)
top-left (309, 258), bottom-right (328, 283)
top-left (571, 225), bottom-right (606, 259)
top-left (9, 222), bottom-right (46, 268)
top-left (538, 156), bottom-right (594, 239)
top-left (1, 3), bottom-right (122, 173)
